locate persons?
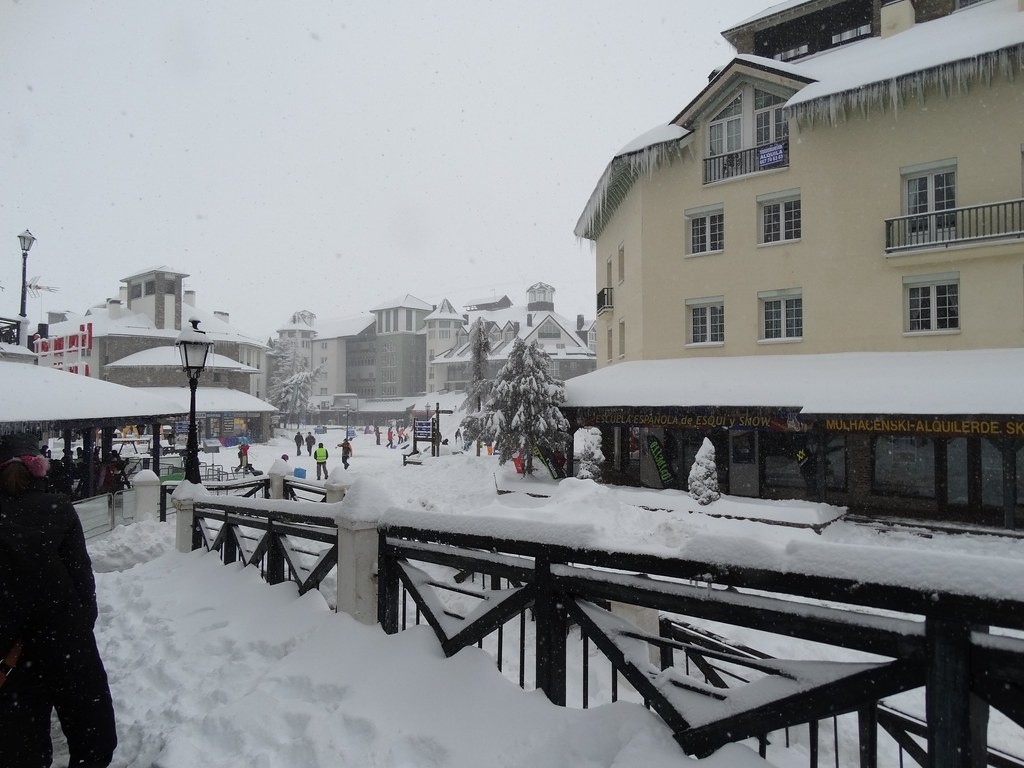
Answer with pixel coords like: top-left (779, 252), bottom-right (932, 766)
top-left (386, 425), bottom-right (406, 448)
top-left (38, 443), bottom-right (82, 464)
top-left (554, 450), bottom-right (566, 469)
top-left (374, 425), bottom-right (382, 445)
top-left (267, 454), bottom-right (292, 478)
top-left (305, 432), bottom-right (315, 456)
top-left (442, 438), bottom-right (449, 446)
top-left (338, 438), bottom-right (352, 469)
top-left (0, 433), bottom-right (117, 768)
top-left (314, 443), bottom-right (328, 480)
top-left (294, 432), bottom-right (304, 456)
top-left (235, 442), bottom-right (255, 473)
top-left (455, 428), bottom-right (462, 445)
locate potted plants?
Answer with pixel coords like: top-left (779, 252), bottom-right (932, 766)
top-left (159, 472), bottom-right (182, 509)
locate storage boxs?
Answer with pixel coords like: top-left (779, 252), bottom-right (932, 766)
top-left (294, 468), bottom-right (307, 478)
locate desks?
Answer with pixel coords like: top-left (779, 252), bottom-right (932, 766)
top-left (113, 438), bottom-right (151, 455)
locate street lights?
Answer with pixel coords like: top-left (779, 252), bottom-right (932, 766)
top-left (16, 228), bottom-right (38, 319)
top-left (345, 403), bottom-right (352, 439)
top-left (424, 403), bottom-right (431, 421)
top-left (175, 317), bottom-right (214, 487)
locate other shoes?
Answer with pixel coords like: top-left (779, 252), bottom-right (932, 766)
top-left (245, 472), bottom-right (249, 474)
top-left (317, 477), bottom-right (320, 480)
top-left (235, 470), bottom-right (238, 474)
top-left (344, 463), bottom-right (349, 469)
top-left (325, 476), bottom-right (328, 479)
